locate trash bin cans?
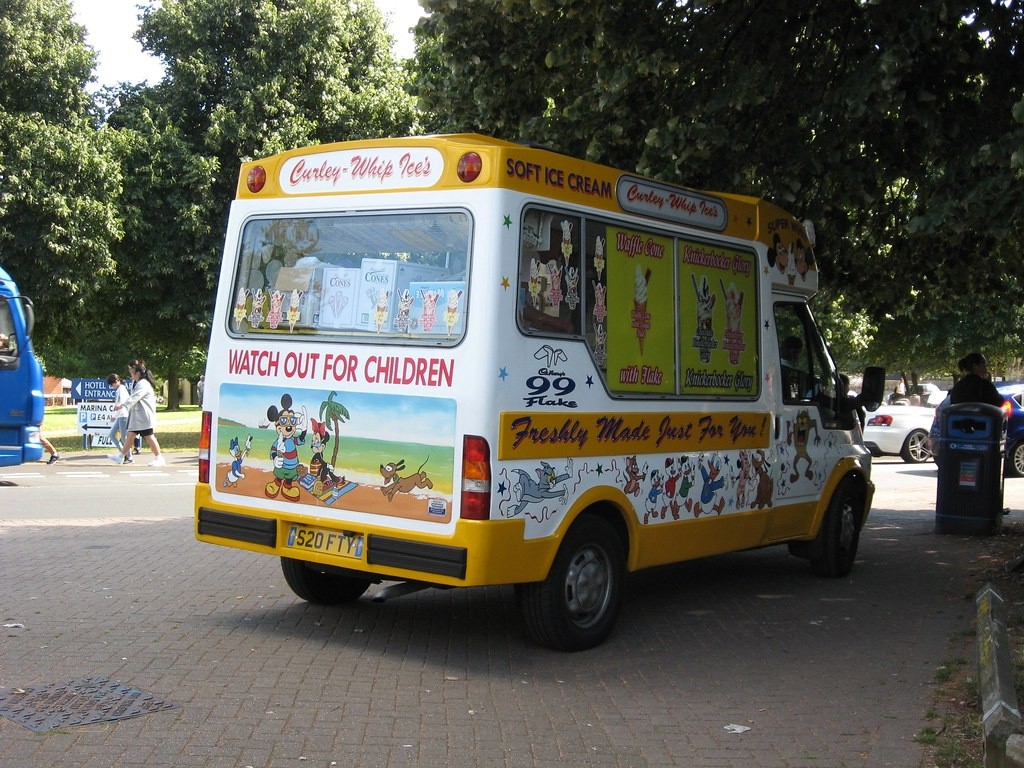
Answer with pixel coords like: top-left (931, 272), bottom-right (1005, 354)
top-left (933, 400), bottom-right (1010, 537)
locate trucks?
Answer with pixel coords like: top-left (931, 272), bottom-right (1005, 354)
top-left (190, 131), bottom-right (884, 652)
top-left (0, 266), bottom-right (60, 467)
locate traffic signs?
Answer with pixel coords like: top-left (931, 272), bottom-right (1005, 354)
top-left (71, 378), bottom-right (134, 399)
top-left (76, 403), bottom-right (121, 433)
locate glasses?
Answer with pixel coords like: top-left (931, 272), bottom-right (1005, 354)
top-left (129, 368), bottom-right (133, 373)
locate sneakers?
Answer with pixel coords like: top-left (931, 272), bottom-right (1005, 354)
top-left (107, 453), bottom-right (123, 464)
top-left (149, 457), bottom-right (165, 466)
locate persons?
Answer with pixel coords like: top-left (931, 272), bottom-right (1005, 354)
top-left (781, 335), bottom-right (830, 407)
top-left (197, 375), bottom-right (205, 408)
top-left (841, 374), bottom-right (865, 431)
top-left (896, 378), bottom-right (905, 397)
top-left (39, 434), bottom-right (61, 466)
top-left (107, 360), bottom-right (165, 466)
top-left (929, 351), bottom-right (1005, 466)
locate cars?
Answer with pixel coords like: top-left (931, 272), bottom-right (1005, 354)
top-left (888, 383), bottom-right (940, 405)
top-left (862, 391), bottom-right (948, 463)
top-left (992, 381), bottom-right (1024, 478)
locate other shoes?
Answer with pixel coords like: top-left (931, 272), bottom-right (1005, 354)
top-left (1003, 508), bottom-right (1010, 515)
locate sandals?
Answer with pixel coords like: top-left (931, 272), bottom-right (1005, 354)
top-left (123, 457), bottom-right (134, 464)
top-left (132, 447), bottom-right (141, 455)
top-left (47, 454), bottom-right (61, 465)
